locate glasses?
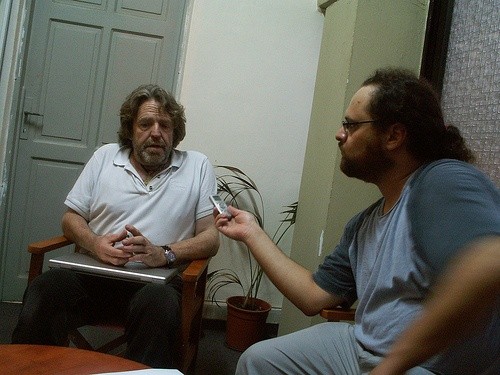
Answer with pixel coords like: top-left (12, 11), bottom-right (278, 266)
top-left (341, 119), bottom-right (395, 133)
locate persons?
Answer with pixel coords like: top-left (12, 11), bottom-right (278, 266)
top-left (12, 84), bottom-right (221, 367)
top-left (213, 66), bottom-right (500, 375)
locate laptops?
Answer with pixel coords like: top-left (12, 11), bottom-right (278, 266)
top-left (50, 252), bottom-right (178, 285)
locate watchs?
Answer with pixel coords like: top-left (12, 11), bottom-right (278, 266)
top-left (160, 245), bottom-right (176, 266)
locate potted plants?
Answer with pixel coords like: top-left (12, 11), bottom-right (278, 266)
top-left (206, 164), bottom-right (298, 352)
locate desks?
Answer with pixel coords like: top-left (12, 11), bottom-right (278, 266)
top-left (0, 343), bottom-right (151, 375)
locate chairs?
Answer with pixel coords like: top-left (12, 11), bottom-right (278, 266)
top-left (28, 236), bottom-right (211, 375)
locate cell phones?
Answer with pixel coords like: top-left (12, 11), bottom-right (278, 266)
top-left (209, 195), bottom-right (232, 218)
